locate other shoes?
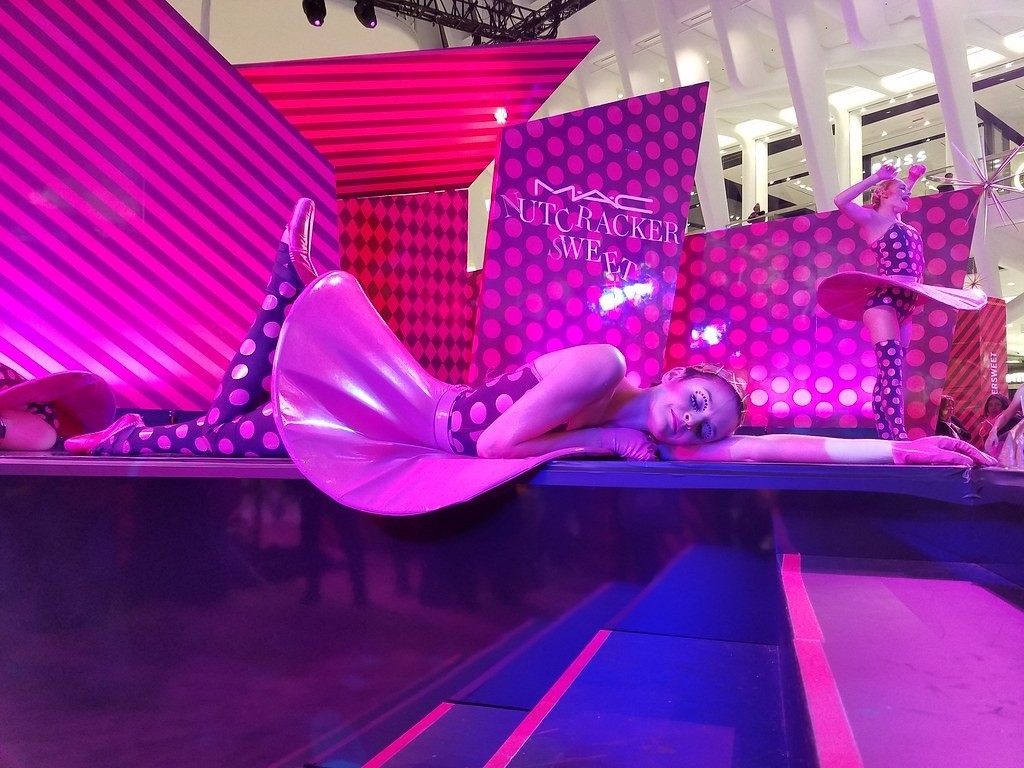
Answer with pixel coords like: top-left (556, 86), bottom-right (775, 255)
top-left (285, 198), bottom-right (319, 287)
top-left (64, 414), bottom-right (145, 453)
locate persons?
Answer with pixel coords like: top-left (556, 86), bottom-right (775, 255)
top-left (0, 366), bottom-right (115, 451)
top-left (937, 173), bottom-right (954, 192)
top-left (816, 165), bottom-right (988, 441)
top-left (979, 383), bottom-right (1024, 467)
top-left (935, 394), bottom-right (972, 442)
top-left (65, 195), bottom-right (998, 517)
top-left (747, 203), bottom-right (765, 223)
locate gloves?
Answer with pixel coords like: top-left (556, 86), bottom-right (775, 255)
top-left (892, 437), bottom-right (999, 465)
top-left (985, 427), bottom-right (999, 452)
top-left (600, 427), bottom-right (659, 462)
